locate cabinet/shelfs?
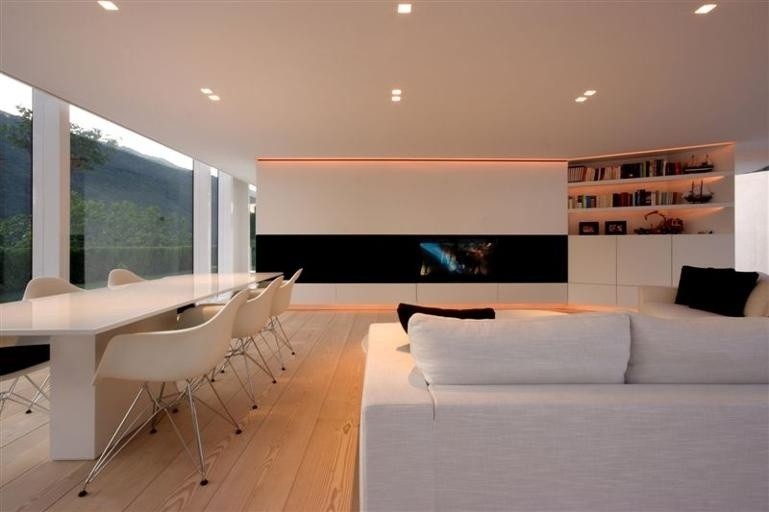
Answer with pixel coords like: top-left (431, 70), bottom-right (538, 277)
top-left (567, 140), bottom-right (736, 309)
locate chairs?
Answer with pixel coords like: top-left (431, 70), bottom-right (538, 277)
top-left (2, 268), bottom-right (303, 498)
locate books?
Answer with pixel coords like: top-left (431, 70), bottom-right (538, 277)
top-left (568, 158), bottom-right (682, 209)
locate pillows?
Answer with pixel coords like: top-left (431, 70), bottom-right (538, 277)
top-left (686, 269), bottom-right (760, 318)
top-left (395, 301), bottom-right (497, 342)
top-left (674, 261), bottom-right (735, 306)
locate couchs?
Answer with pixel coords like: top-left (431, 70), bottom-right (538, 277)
top-left (357, 271), bottom-right (769, 511)
